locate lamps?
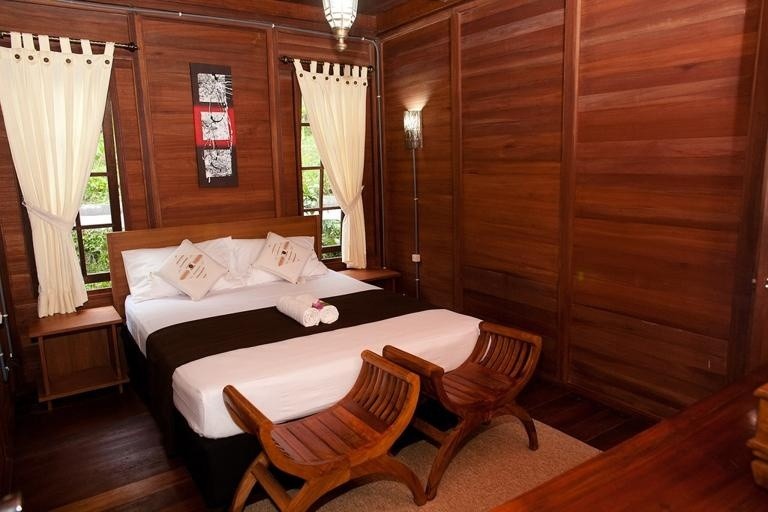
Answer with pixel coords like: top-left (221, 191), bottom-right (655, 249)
top-left (322, 0), bottom-right (360, 56)
top-left (402, 107), bottom-right (423, 297)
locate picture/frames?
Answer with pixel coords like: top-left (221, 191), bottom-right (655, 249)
top-left (195, 146), bottom-right (239, 190)
top-left (191, 106), bottom-right (237, 147)
top-left (187, 61), bottom-right (234, 106)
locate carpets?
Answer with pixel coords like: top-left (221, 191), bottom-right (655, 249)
top-left (233, 407), bottom-right (606, 511)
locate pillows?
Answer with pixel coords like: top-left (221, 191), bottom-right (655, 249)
top-left (251, 230), bottom-right (315, 285)
top-left (230, 234), bottom-right (329, 286)
top-left (119, 235), bottom-right (242, 304)
top-left (153, 238), bottom-right (231, 302)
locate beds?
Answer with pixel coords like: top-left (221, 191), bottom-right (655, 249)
top-left (102, 213), bottom-right (497, 512)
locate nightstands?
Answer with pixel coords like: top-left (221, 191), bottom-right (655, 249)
top-left (336, 267), bottom-right (399, 291)
top-left (26, 304), bottom-right (130, 409)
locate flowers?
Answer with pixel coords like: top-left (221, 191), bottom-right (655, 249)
top-left (311, 299), bottom-right (329, 310)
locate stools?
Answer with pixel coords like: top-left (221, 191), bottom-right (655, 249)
top-left (221, 347), bottom-right (429, 512)
top-left (381, 320), bottom-right (542, 503)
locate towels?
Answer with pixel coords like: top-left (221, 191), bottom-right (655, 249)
top-left (276, 295), bottom-right (320, 327)
top-left (295, 292), bottom-right (338, 325)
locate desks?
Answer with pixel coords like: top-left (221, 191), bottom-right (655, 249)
top-left (491, 368), bottom-right (766, 512)
